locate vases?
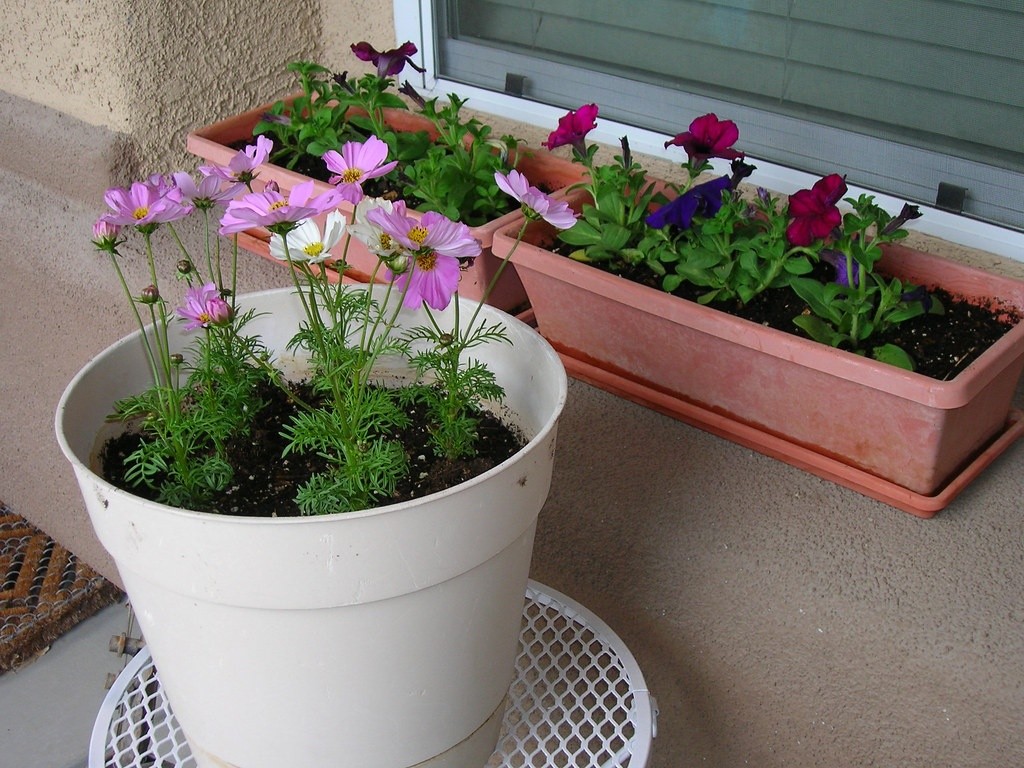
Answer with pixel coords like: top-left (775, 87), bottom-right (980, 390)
top-left (183, 91), bottom-right (604, 313)
top-left (488, 172), bottom-right (1024, 497)
top-left (51, 283), bottom-right (567, 768)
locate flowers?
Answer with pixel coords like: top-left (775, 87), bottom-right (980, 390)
top-left (252, 42), bottom-right (534, 224)
top-left (90, 134), bottom-right (578, 517)
top-left (539, 101), bottom-right (946, 376)
